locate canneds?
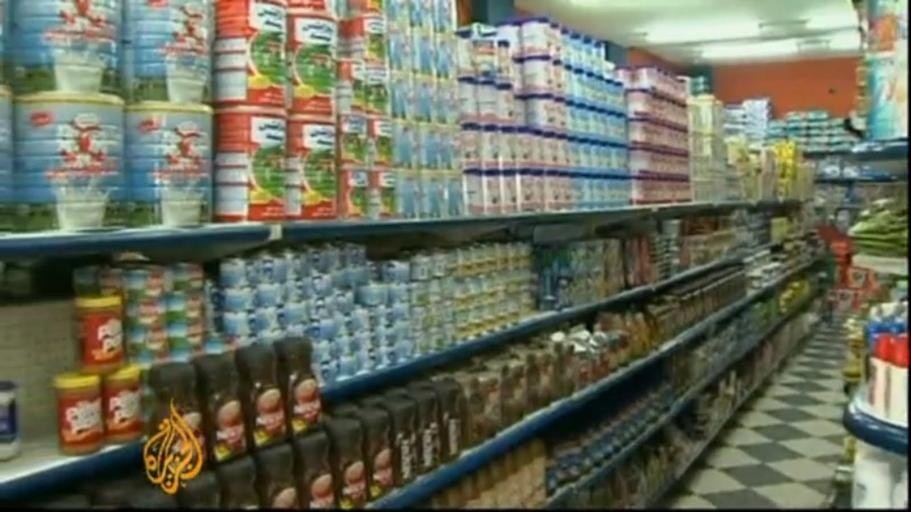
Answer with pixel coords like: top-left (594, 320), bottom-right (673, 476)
top-left (2, 2), bottom-right (466, 231)
top-left (49, 238), bottom-right (538, 456)
top-left (455, 16), bottom-right (695, 220)
